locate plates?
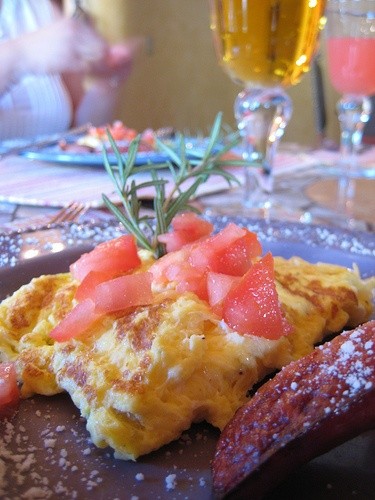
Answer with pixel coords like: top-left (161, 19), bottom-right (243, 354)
top-left (20, 139), bottom-right (226, 164)
top-left (1, 214), bottom-right (375, 500)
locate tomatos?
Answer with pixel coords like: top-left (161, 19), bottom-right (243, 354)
top-left (1, 211), bottom-right (290, 406)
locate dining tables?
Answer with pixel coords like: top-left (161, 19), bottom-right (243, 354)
top-left (0, 130), bottom-right (375, 500)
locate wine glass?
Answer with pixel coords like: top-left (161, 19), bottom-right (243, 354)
top-left (315, 1), bottom-right (375, 178)
top-left (198, 0), bottom-right (327, 211)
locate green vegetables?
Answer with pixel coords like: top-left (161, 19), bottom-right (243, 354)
top-left (98, 111), bottom-right (262, 254)
